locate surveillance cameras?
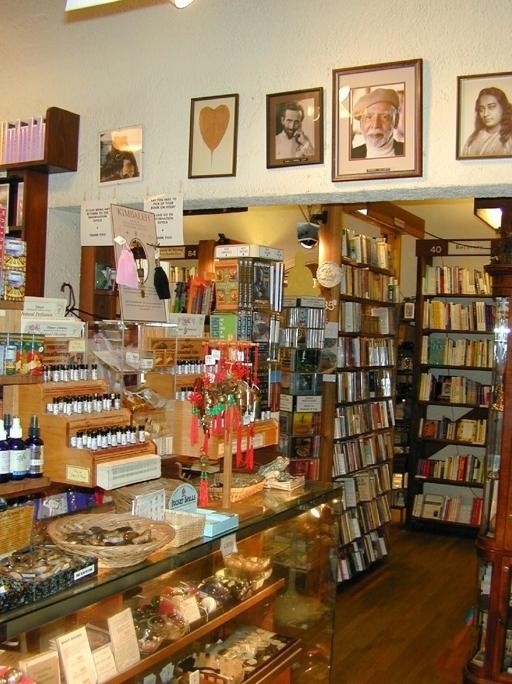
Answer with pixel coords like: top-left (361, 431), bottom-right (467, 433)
top-left (295, 222), bottom-right (321, 249)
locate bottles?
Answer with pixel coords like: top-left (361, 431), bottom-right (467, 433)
top-left (45, 363), bottom-right (98, 382)
top-left (73, 422), bottom-right (149, 452)
top-left (0, 413), bottom-right (45, 481)
top-left (48, 392), bottom-right (121, 416)
top-left (176, 359), bottom-right (205, 375)
top-left (180, 387), bottom-right (193, 401)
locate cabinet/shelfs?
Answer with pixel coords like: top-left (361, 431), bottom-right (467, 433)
top-left (459, 195), bottom-right (511, 679)
top-left (394, 291), bottom-right (418, 513)
top-left (0, 191), bottom-right (346, 684)
top-left (310, 200), bottom-right (425, 592)
top-left (415, 235), bottom-right (496, 537)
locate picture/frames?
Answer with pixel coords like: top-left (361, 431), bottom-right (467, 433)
top-left (98, 122), bottom-right (143, 188)
top-left (455, 71), bottom-right (512, 161)
top-left (330, 58), bottom-right (423, 183)
top-left (265, 87), bottom-right (324, 169)
top-left (187, 92), bottom-right (239, 180)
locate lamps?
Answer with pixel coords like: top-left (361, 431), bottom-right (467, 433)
top-left (169, 0), bottom-right (193, 9)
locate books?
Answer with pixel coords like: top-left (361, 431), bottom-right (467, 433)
top-left (332, 229), bottom-right (398, 585)
top-left (412, 263), bottom-right (493, 527)
top-left (5, 114), bottom-right (46, 164)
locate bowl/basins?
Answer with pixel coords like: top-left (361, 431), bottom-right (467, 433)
top-left (145, 613), bottom-right (189, 639)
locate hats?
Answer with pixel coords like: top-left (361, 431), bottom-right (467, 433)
top-left (352, 89), bottom-right (400, 120)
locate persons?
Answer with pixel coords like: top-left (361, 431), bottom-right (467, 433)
top-left (275, 101), bottom-right (314, 160)
top-left (463, 86), bottom-right (512, 157)
top-left (351, 88), bottom-right (405, 158)
